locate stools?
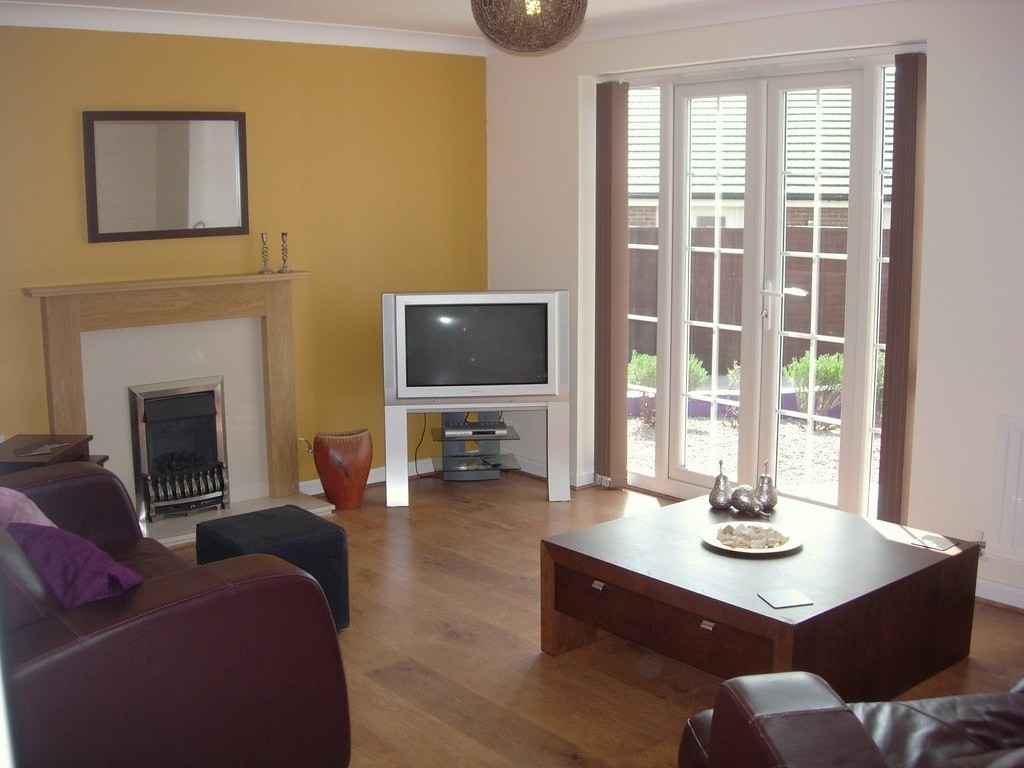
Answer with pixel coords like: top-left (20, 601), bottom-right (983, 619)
top-left (198, 504), bottom-right (350, 632)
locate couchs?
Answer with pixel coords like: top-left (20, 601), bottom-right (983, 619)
top-left (677, 670), bottom-right (1024, 768)
top-left (0, 462), bottom-right (352, 768)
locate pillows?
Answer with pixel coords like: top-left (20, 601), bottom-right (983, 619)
top-left (0, 485), bottom-right (145, 612)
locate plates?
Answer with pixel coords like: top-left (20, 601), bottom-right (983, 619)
top-left (703, 522), bottom-right (802, 554)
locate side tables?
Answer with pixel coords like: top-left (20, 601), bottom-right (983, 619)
top-left (0, 433), bottom-right (111, 471)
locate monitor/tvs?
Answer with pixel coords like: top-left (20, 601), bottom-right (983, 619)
top-left (396, 293), bottom-right (557, 397)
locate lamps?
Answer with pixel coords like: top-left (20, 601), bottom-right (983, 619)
top-left (471, 0), bottom-right (588, 53)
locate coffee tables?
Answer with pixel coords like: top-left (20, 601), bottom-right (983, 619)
top-left (538, 491), bottom-right (982, 703)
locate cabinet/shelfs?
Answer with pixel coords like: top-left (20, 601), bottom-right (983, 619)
top-left (429, 413), bottom-right (523, 480)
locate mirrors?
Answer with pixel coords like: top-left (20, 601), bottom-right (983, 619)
top-left (82, 111), bottom-right (250, 244)
